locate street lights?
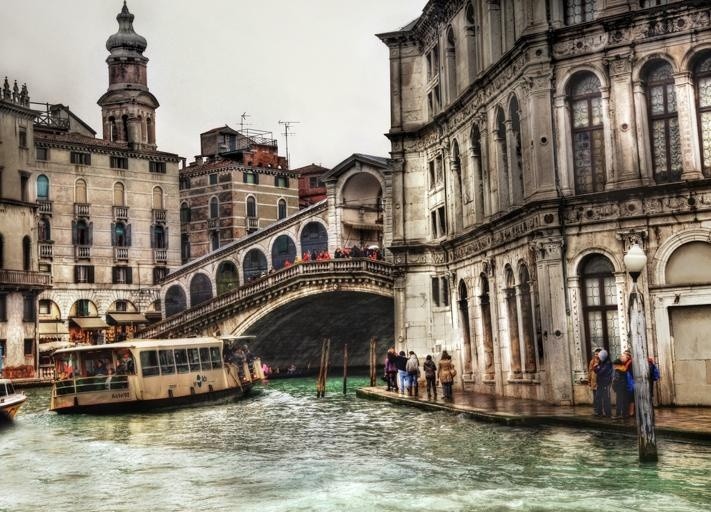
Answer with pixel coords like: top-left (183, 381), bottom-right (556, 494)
top-left (624, 244), bottom-right (658, 460)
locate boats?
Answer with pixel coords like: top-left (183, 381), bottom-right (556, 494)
top-left (48, 334), bottom-right (264, 413)
top-left (0, 378), bottom-right (29, 424)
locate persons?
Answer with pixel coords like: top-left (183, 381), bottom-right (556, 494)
top-left (283, 244), bottom-right (385, 267)
top-left (588, 348), bottom-right (660, 419)
top-left (381, 348), bottom-right (457, 399)
top-left (55, 354), bottom-right (134, 381)
top-left (224, 344), bottom-right (273, 383)
top-left (287, 362), bottom-right (297, 376)
top-left (247, 269), bottom-right (275, 284)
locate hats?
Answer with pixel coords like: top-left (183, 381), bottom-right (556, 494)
top-left (598, 350), bottom-right (608, 362)
